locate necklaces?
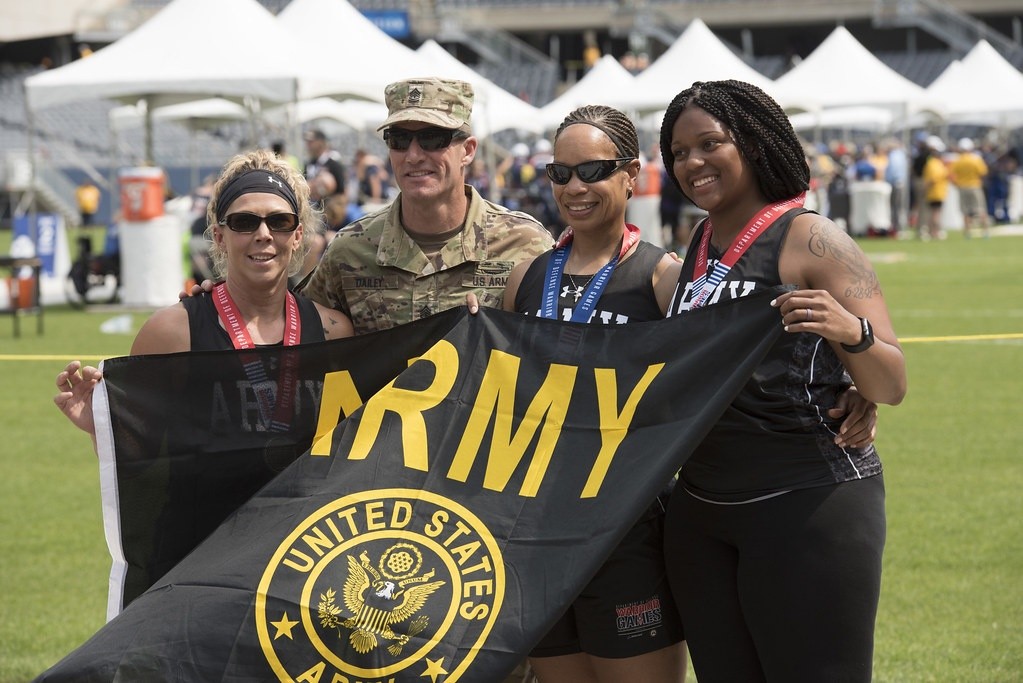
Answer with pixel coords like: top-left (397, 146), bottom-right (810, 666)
top-left (567, 251), bottom-right (619, 302)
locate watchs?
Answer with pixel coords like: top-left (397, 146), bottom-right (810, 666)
top-left (840, 317), bottom-right (874, 353)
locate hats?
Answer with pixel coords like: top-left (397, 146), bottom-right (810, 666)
top-left (376, 77), bottom-right (474, 131)
top-left (958, 137), bottom-right (974, 151)
top-left (303, 130), bottom-right (326, 141)
top-left (927, 135), bottom-right (946, 152)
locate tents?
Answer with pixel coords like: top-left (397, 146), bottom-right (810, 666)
top-left (23, 0), bottom-right (550, 304)
top-left (546, 17), bottom-right (1023, 154)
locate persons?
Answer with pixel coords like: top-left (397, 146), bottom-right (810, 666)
top-left (55, 155), bottom-right (354, 454)
top-left (187, 132), bottom-right (1023, 286)
top-left (555, 79), bottom-right (909, 683)
top-left (178, 79), bottom-right (683, 683)
top-left (467, 104), bottom-right (878, 683)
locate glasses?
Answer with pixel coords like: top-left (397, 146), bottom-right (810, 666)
top-left (546, 157), bottom-right (640, 186)
top-left (383, 126), bottom-right (471, 151)
top-left (217, 211), bottom-right (300, 232)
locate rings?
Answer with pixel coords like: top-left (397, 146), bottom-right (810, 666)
top-left (807, 309), bottom-right (811, 320)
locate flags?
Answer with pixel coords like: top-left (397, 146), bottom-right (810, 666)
top-left (32, 303), bottom-right (782, 683)
